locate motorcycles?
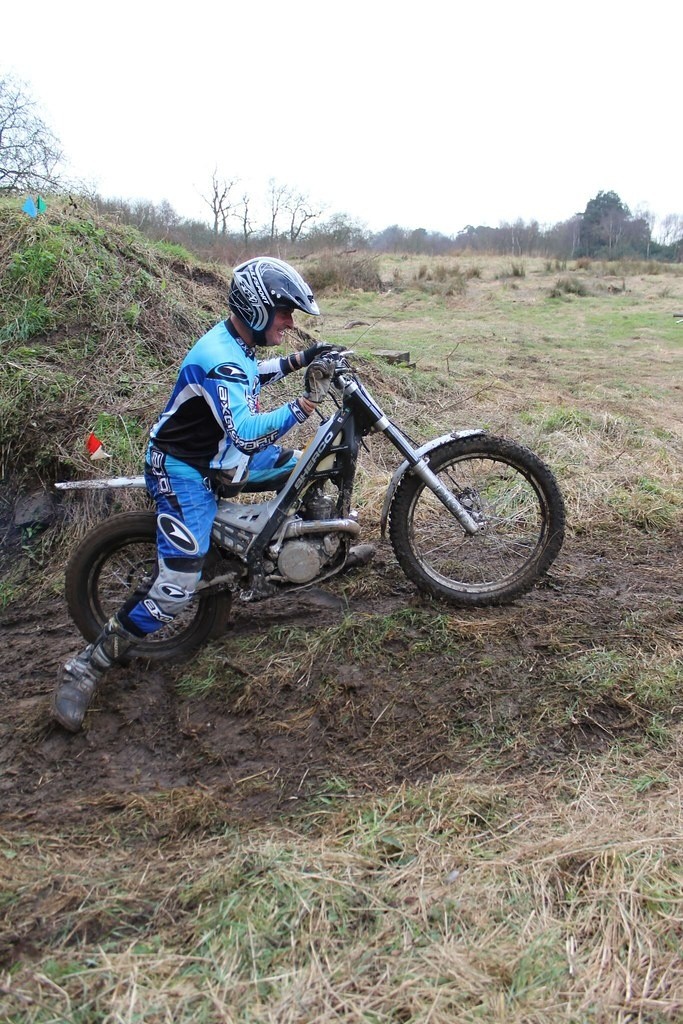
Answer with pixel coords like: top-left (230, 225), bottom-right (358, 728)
top-left (54, 348), bottom-right (565, 673)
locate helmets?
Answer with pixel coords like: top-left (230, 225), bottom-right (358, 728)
top-left (228, 257), bottom-right (321, 332)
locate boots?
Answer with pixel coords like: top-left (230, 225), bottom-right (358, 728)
top-left (52, 612), bottom-right (148, 733)
top-left (345, 544), bottom-right (378, 567)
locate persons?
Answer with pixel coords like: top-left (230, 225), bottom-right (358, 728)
top-left (53, 256), bottom-right (348, 734)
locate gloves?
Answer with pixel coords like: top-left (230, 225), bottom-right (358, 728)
top-left (300, 358), bottom-right (334, 403)
top-left (299, 342), bottom-right (347, 367)
